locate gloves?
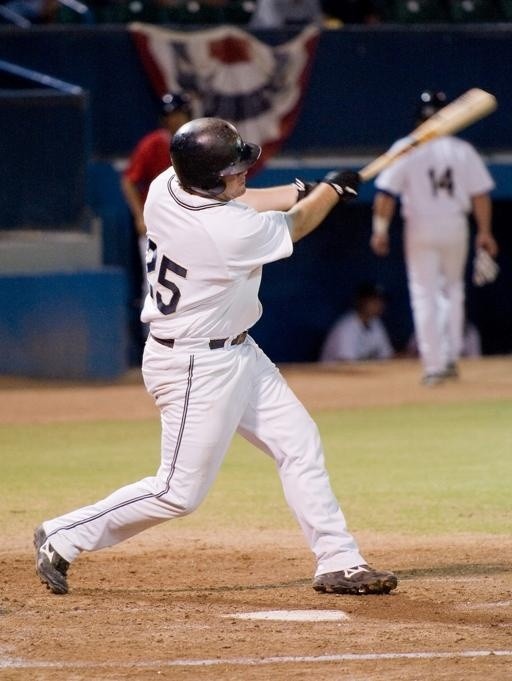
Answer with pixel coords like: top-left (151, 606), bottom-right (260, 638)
top-left (321, 168), bottom-right (363, 201)
top-left (296, 176), bottom-right (321, 204)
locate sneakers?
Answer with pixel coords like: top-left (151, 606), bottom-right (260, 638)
top-left (32, 524), bottom-right (71, 595)
top-left (312, 564), bottom-right (398, 598)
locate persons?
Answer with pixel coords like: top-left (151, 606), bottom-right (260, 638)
top-left (368, 84), bottom-right (498, 378)
top-left (32, 116), bottom-right (400, 598)
top-left (316, 289), bottom-right (398, 363)
top-left (406, 315), bottom-right (482, 357)
top-left (120, 90), bottom-right (198, 335)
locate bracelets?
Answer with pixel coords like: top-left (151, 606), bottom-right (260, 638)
top-left (371, 215), bottom-right (391, 236)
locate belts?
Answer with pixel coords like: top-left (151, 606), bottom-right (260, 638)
top-left (150, 330), bottom-right (250, 352)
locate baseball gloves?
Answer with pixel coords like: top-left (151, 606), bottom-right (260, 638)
top-left (475, 249), bottom-right (497, 285)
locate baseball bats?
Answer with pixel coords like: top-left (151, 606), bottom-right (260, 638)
top-left (356, 88), bottom-right (497, 182)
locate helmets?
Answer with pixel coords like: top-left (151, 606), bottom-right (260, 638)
top-left (168, 115), bottom-right (273, 198)
